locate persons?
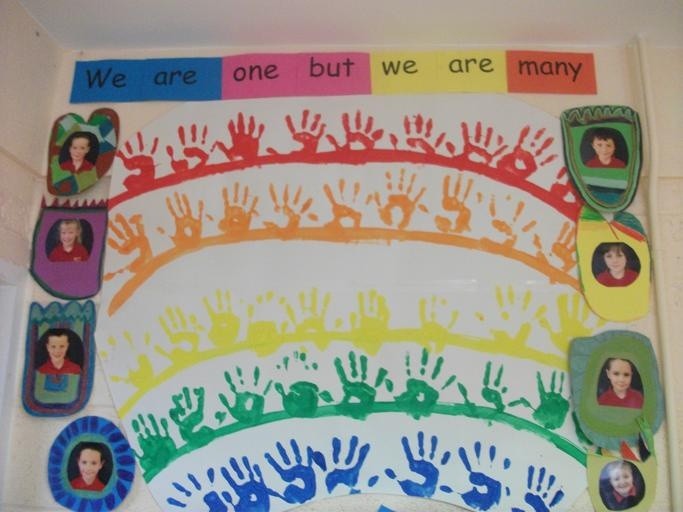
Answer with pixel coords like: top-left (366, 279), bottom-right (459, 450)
top-left (61, 133), bottom-right (94, 172)
top-left (596, 244), bottom-right (639, 287)
top-left (37, 330), bottom-right (82, 373)
top-left (604, 462), bottom-right (643, 510)
top-left (584, 130), bottom-right (625, 168)
top-left (70, 444), bottom-right (108, 491)
top-left (597, 358), bottom-right (643, 409)
top-left (48, 220), bottom-right (89, 261)
